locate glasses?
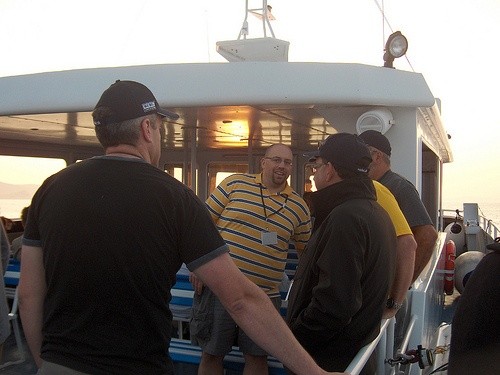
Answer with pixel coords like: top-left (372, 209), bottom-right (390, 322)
top-left (263, 154), bottom-right (294, 166)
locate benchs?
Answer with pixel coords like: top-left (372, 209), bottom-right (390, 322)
top-left (4, 213), bottom-right (316, 368)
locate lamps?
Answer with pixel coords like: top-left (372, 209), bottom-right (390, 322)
top-left (382, 31), bottom-right (408, 69)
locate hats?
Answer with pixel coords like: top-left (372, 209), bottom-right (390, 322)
top-left (92, 79), bottom-right (180, 125)
top-left (359, 130), bottom-right (391, 156)
top-left (307, 132), bottom-right (374, 186)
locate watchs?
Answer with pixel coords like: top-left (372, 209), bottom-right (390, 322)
top-left (385, 297), bottom-right (403, 311)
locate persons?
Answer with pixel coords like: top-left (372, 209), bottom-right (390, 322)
top-left (371, 179), bottom-right (418, 320)
top-left (358, 129), bottom-right (438, 290)
top-left (12, 205), bottom-right (29, 260)
top-left (189, 142), bottom-right (313, 375)
top-left (18, 80), bottom-right (350, 375)
top-left (283, 132), bottom-right (398, 375)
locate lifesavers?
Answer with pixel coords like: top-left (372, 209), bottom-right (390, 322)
top-left (445, 240), bottom-right (456, 295)
